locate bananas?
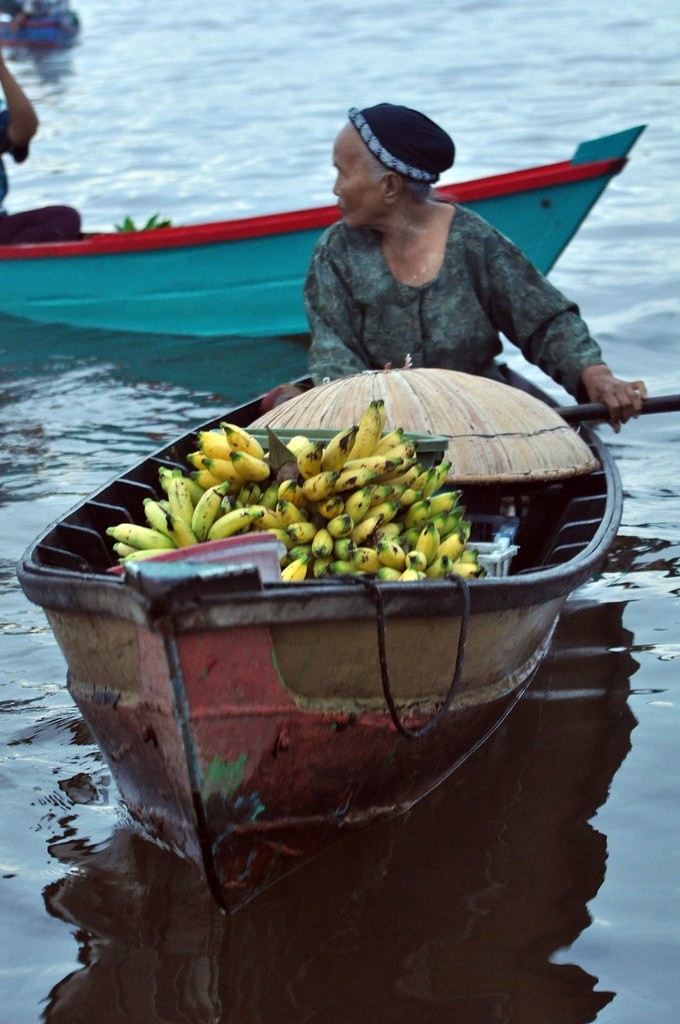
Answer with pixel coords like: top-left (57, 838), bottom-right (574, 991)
top-left (100, 395), bottom-right (482, 584)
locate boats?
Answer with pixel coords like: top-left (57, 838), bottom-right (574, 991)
top-left (1, 1), bottom-right (82, 51)
top-left (0, 124), bottom-right (647, 340)
top-left (13, 356), bottom-right (626, 915)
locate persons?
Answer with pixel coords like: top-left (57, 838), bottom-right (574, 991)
top-left (0, 0), bottom-right (81, 246)
top-left (260, 102), bottom-right (647, 433)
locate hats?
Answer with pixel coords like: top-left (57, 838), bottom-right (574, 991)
top-left (348, 103), bottom-right (456, 183)
